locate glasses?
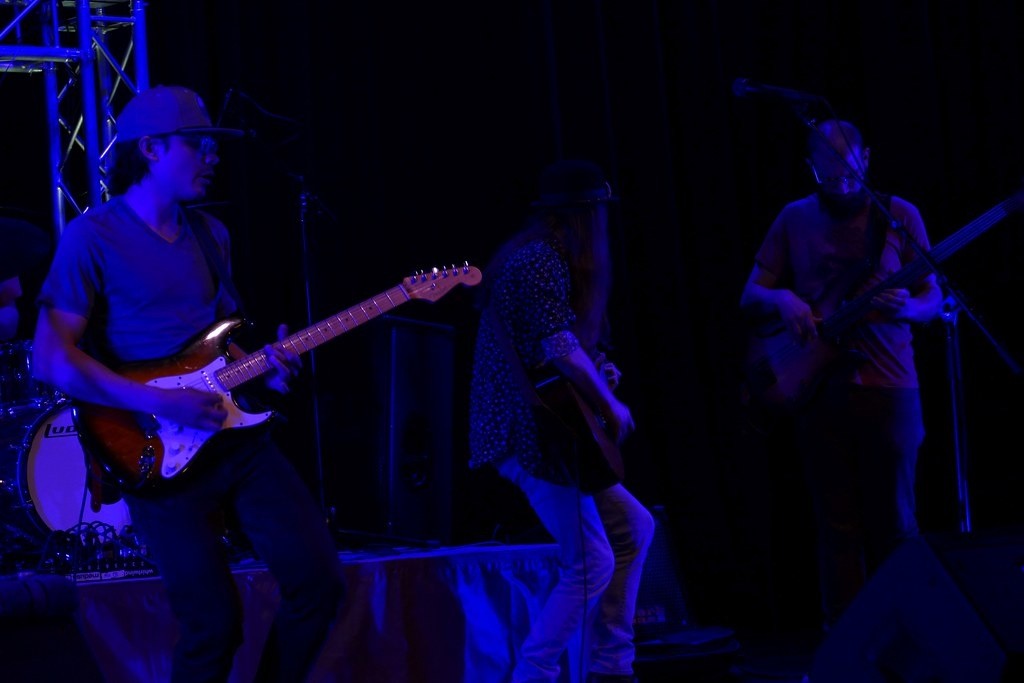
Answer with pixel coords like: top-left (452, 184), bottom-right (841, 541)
top-left (177, 132), bottom-right (218, 156)
top-left (812, 161), bottom-right (866, 189)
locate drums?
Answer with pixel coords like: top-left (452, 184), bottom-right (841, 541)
top-left (8, 391), bottom-right (133, 550)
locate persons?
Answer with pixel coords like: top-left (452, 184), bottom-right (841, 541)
top-left (740, 118), bottom-right (944, 639)
top-left (468, 150), bottom-right (656, 683)
top-left (35, 84), bottom-right (348, 683)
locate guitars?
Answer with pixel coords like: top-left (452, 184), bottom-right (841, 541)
top-left (79, 260), bottom-right (484, 489)
top-left (534, 361), bottom-right (625, 491)
top-left (743, 188), bottom-right (1024, 407)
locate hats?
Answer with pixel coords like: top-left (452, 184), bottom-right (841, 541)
top-left (113, 84), bottom-right (244, 145)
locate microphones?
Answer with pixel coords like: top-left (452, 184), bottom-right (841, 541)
top-left (733, 78), bottom-right (826, 103)
